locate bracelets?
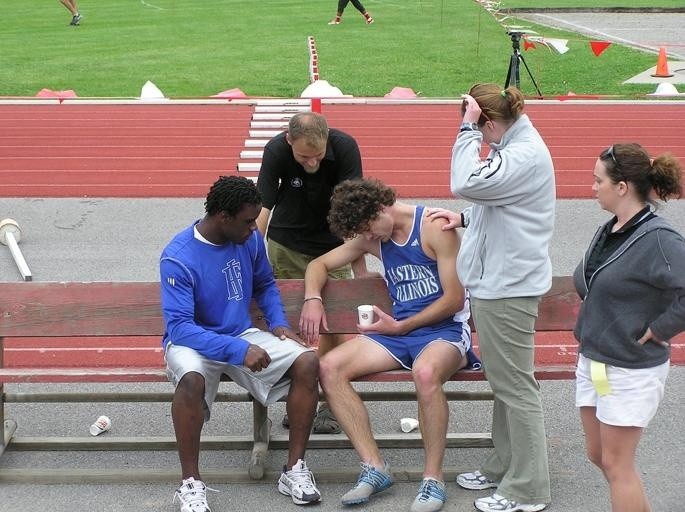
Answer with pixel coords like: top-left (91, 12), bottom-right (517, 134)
top-left (304, 295), bottom-right (322, 301)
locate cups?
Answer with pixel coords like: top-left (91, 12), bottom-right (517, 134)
top-left (398, 416), bottom-right (419, 436)
top-left (357, 304), bottom-right (376, 325)
top-left (88, 413), bottom-right (112, 436)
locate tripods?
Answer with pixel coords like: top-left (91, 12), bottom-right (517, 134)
top-left (504, 36), bottom-right (544, 100)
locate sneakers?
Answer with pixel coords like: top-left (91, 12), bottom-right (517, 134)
top-left (313, 402), bottom-right (342, 433)
top-left (70, 13), bottom-right (82, 25)
top-left (328, 20), bottom-right (340, 24)
top-left (366, 18), bottom-right (374, 24)
top-left (175, 477), bottom-right (212, 512)
top-left (282, 414), bottom-right (290, 430)
top-left (456, 470), bottom-right (499, 491)
top-left (278, 459), bottom-right (322, 505)
top-left (411, 479), bottom-right (447, 511)
top-left (473, 493), bottom-right (548, 512)
top-left (341, 461), bottom-right (393, 505)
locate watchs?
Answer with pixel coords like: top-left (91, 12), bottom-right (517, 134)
top-left (459, 122), bottom-right (480, 130)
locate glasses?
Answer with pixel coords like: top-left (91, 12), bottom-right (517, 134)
top-left (600, 145), bottom-right (628, 188)
top-left (462, 83), bottom-right (494, 126)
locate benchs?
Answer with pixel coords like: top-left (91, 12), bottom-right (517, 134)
top-left (0, 275), bottom-right (684, 482)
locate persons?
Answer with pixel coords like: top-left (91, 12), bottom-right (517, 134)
top-left (328, 0), bottom-right (374, 25)
top-left (426, 84), bottom-right (556, 512)
top-left (60, 0), bottom-right (83, 26)
top-left (255, 113), bottom-right (379, 435)
top-left (298, 178), bottom-right (482, 512)
top-left (159, 176), bottom-right (322, 512)
top-left (572, 143), bottom-right (685, 512)
